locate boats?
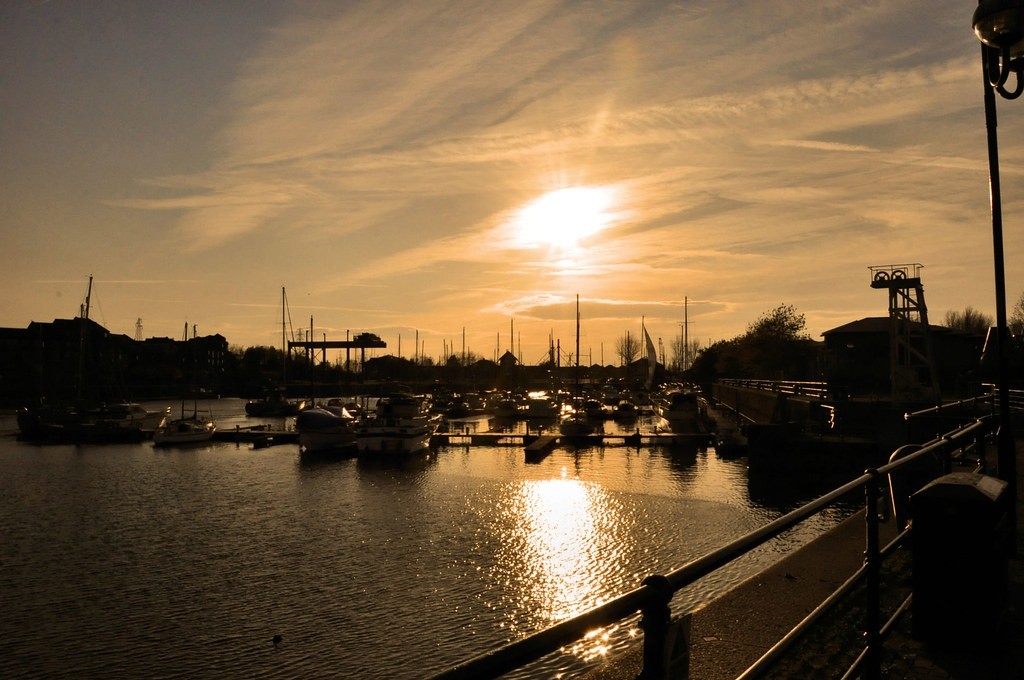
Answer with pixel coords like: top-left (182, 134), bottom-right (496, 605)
top-left (353, 392), bottom-right (432, 456)
top-left (240, 377), bottom-right (298, 418)
top-left (292, 407), bottom-right (358, 455)
top-left (286, 393), bottom-right (370, 425)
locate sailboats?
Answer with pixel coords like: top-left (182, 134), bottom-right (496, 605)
top-left (421, 303), bottom-right (725, 437)
top-left (16, 275), bottom-right (219, 448)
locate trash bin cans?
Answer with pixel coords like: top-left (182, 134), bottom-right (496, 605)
top-left (910, 473), bottom-right (1010, 638)
